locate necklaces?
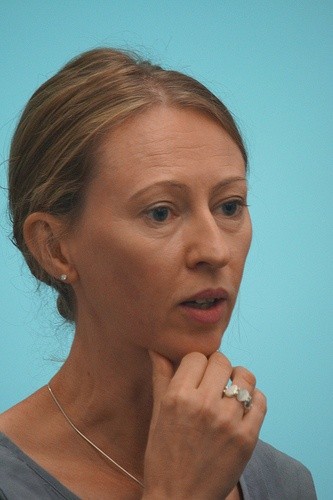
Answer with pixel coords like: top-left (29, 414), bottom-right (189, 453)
top-left (47, 383), bottom-right (144, 489)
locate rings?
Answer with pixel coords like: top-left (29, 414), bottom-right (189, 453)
top-left (223, 384), bottom-right (254, 414)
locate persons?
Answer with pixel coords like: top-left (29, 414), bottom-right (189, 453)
top-left (0, 42), bottom-right (317, 500)
